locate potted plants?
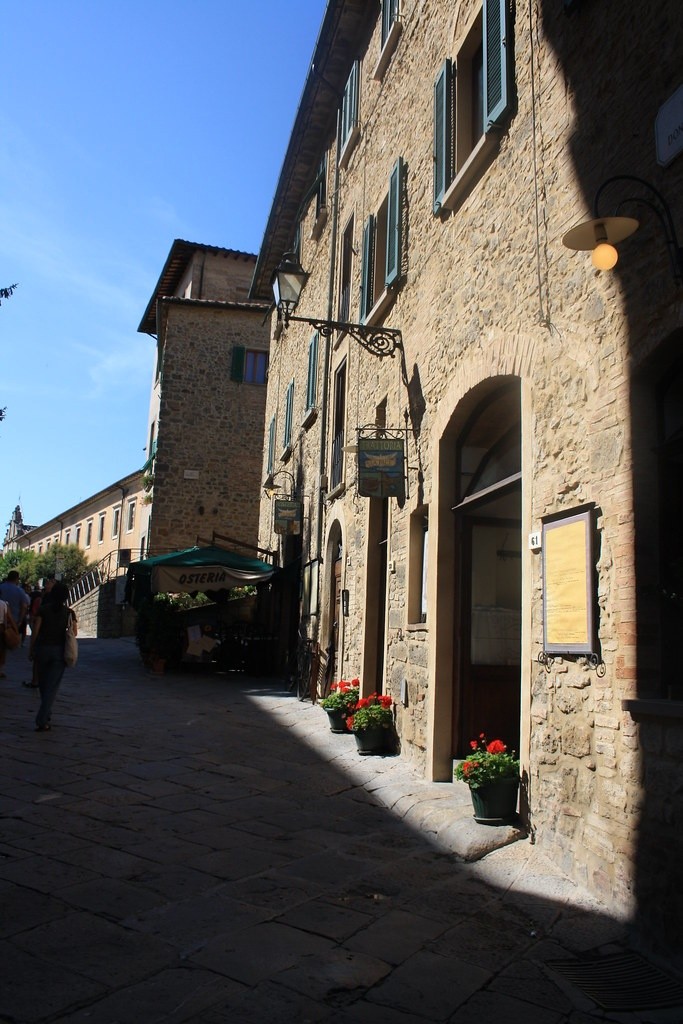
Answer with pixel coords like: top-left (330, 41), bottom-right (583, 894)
top-left (134, 583), bottom-right (258, 668)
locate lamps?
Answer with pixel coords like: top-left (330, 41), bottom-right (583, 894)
top-left (262, 470), bottom-right (304, 498)
top-left (339, 422), bottom-right (422, 465)
top-left (268, 248), bottom-right (403, 360)
top-left (562, 173), bottom-right (683, 288)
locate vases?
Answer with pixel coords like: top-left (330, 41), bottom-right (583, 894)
top-left (324, 708), bottom-right (354, 730)
top-left (467, 779), bottom-right (518, 819)
top-left (353, 729), bottom-right (394, 752)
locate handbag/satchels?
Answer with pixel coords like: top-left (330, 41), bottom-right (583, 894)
top-left (4, 627), bottom-right (20, 650)
top-left (64, 610), bottom-right (78, 668)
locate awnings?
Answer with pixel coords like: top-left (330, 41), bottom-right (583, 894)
top-left (126, 545), bottom-right (282, 594)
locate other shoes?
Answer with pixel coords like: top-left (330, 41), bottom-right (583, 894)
top-left (47, 710), bottom-right (52, 720)
top-left (39, 724), bottom-right (49, 730)
top-left (0, 671), bottom-right (8, 677)
top-left (22, 680), bottom-right (39, 689)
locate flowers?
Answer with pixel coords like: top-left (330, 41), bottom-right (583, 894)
top-left (340, 690), bottom-right (392, 731)
top-left (455, 732), bottom-right (520, 789)
top-left (320, 679), bottom-right (360, 711)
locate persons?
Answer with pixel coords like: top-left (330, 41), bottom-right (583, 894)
top-left (0, 571), bottom-right (77, 730)
